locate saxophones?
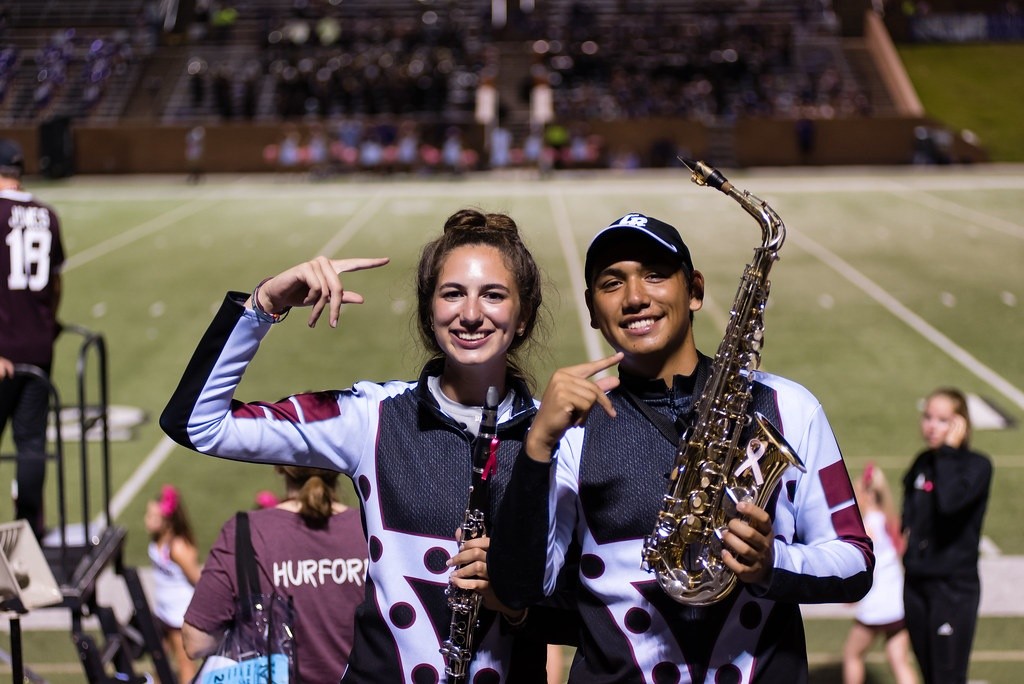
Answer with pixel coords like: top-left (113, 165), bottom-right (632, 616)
top-left (637, 148), bottom-right (812, 612)
top-left (439, 383), bottom-right (502, 684)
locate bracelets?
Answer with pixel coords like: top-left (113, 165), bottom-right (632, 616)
top-left (251, 277), bottom-right (293, 324)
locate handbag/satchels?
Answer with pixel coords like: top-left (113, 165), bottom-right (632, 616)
top-left (191, 512), bottom-right (299, 684)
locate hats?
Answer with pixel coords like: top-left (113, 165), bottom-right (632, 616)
top-left (585, 213), bottom-right (694, 290)
top-left (0, 140), bottom-right (23, 166)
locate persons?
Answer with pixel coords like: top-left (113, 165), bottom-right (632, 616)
top-left (144, 390), bottom-right (368, 684)
top-left (159, 209), bottom-right (581, 684)
top-left (844, 460), bottom-right (920, 684)
top-left (900, 388), bottom-right (993, 684)
top-left (0, 138), bottom-right (66, 550)
top-left (486, 213), bottom-right (875, 684)
